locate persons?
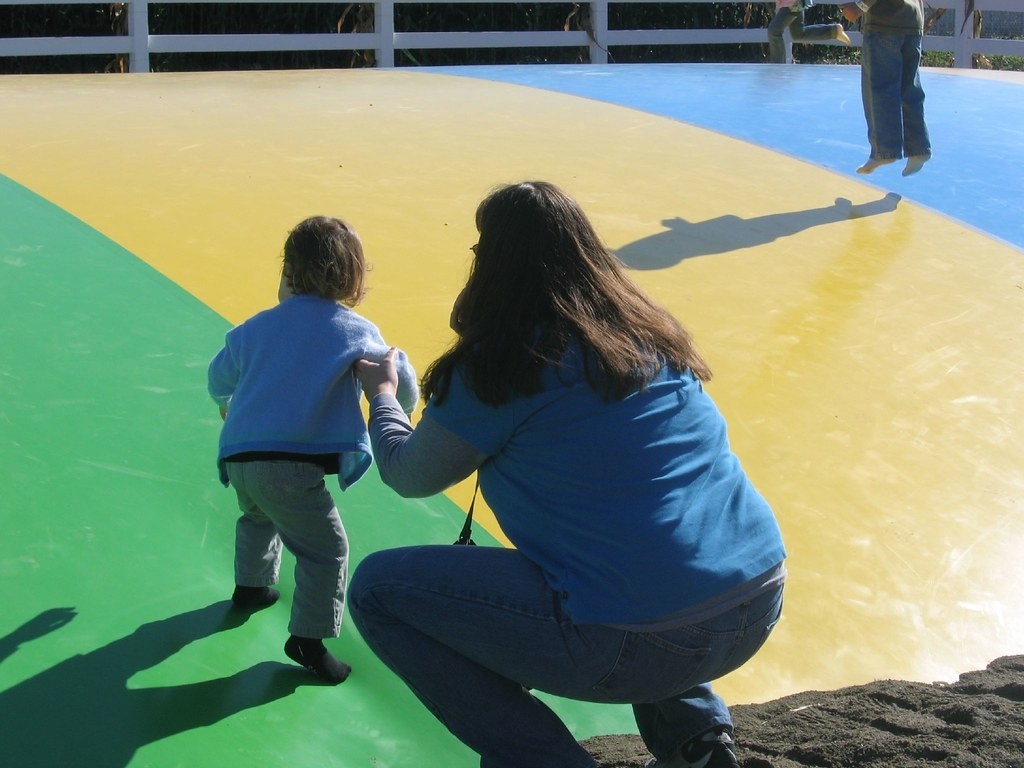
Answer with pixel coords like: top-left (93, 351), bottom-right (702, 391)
top-left (346, 183), bottom-right (788, 768)
top-left (768, 0), bottom-right (934, 179)
top-left (206, 216), bottom-right (421, 682)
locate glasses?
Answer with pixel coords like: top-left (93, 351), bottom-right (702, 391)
top-left (470, 244), bottom-right (478, 255)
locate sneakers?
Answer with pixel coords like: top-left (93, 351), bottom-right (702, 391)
top-left (644, 725), bottom-right (741, 768)
